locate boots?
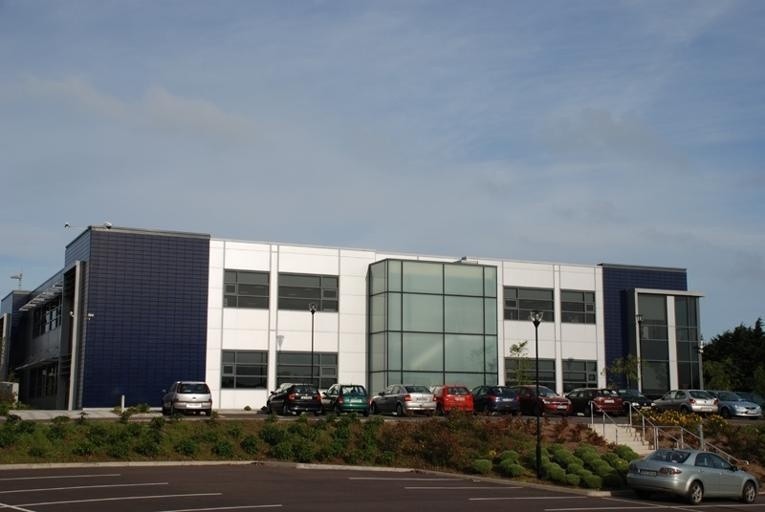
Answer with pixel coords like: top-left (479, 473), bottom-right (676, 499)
top-left (158, 379), bottom-right (211, 417)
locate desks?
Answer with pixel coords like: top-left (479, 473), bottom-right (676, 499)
top-left (634, 313), bottom-right (646, 396)
top-left (9, 272), bottom-right (22, 289)
top-left (308, 300), bottom-right (316, 383)
top-left (526, 307), bottom-right (545, 479)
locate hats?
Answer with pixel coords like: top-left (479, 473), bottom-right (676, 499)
top-left (651, 387), bottom-right (764, 421)
top-left (624, 447), bottom-right (760, 506)
